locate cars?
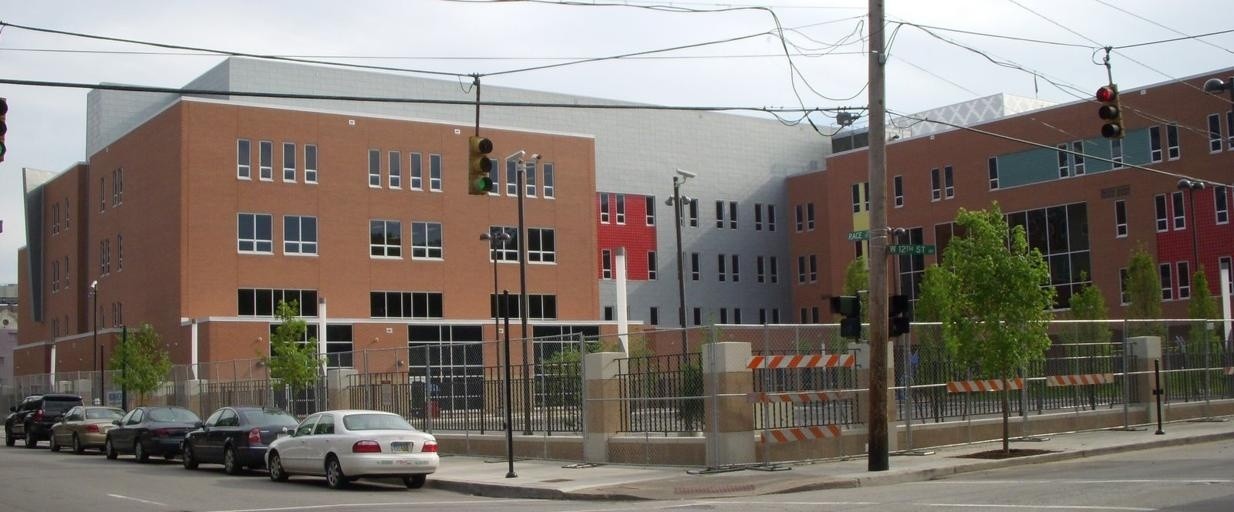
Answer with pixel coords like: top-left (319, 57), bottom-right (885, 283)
top-left (182, 404), bottom-right (302, 476)
top-left (105, 405), bottom-right (203, 463)
top-left (48, 406), bottom-right (127, 455)
top-left (265, 408), bottom-right (440, 490)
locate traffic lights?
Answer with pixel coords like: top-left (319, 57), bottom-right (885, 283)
top-left (830, 294), bottom-right (859, 338)
top-left (468, 135), bottom-right (493, 196)
top-left (0, 97), bottom-right (8, 162)
top-left (888, 294), bottom-right (910, 338)
top-left (1096, 83), bottom-right (1125, 140)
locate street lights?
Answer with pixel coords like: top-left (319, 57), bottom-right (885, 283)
top-left (478, 232), bottom-right (519, 478)
top-left (1176, 178), bottom-right (1206, 271)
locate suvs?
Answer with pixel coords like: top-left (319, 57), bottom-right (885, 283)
top-left (4, 392), bottom-right (85, 449)
